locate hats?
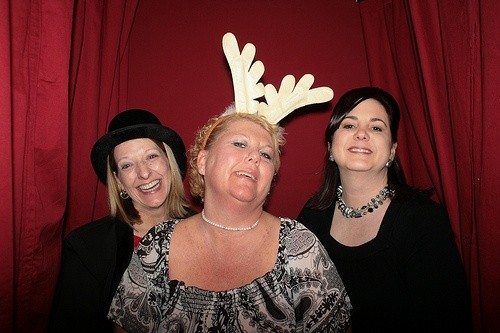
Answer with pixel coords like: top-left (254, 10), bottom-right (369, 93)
top-left (90, 109), bottom-right (186, 187)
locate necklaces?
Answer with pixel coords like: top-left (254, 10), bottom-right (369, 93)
top-left (201, 209), bottom-right (261, 233)
top-left (336, 182), bottom-right (395, 219)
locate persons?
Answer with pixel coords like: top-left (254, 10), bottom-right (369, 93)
top-left (51, 109), bottom-right (199, 333)
top-left (107, 106), bottom-right (352, 333)
top-left (295, 88), bottom-right (473, 333)
